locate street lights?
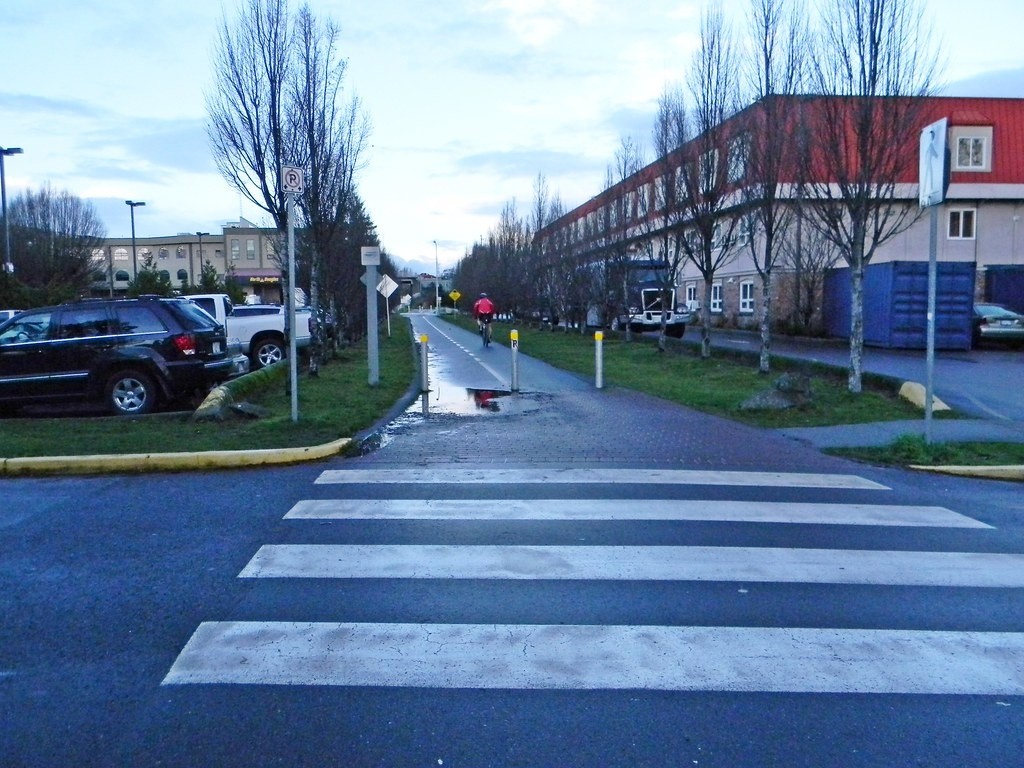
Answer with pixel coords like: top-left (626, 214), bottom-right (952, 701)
top-left (433, 241), bottom-right (438, 313)
top-left (0, 148), bottom-right (23, 273)
top-left (125, 200), bottom-right (145, 282)
top-left (196, 232), bottom-right (209, 278)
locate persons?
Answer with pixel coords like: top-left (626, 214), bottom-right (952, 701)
top-left (474, 293), bottom-right (494, 338)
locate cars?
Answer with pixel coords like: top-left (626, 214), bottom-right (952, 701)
top-left (973, 302), bottom-right (1024, 348)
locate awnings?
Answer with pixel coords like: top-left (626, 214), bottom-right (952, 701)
top-left (225, 267), bottom-right (282, 283)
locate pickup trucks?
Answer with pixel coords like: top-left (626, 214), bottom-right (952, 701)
top-left (174, 294), bottom-right (312, 368)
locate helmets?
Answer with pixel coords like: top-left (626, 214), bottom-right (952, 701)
top-left (481, 292), bottom-right (487, 298)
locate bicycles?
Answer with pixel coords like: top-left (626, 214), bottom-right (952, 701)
top-left (475, 314), bottom-right (489, 347)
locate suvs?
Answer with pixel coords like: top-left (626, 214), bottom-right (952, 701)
top-left (0, 294), bottom-right (250, 414)
top-left (516, 296), bottom-right (559, 325)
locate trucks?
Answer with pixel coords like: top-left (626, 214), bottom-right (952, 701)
top-left (565, 259), bottom-right (690, 338)
